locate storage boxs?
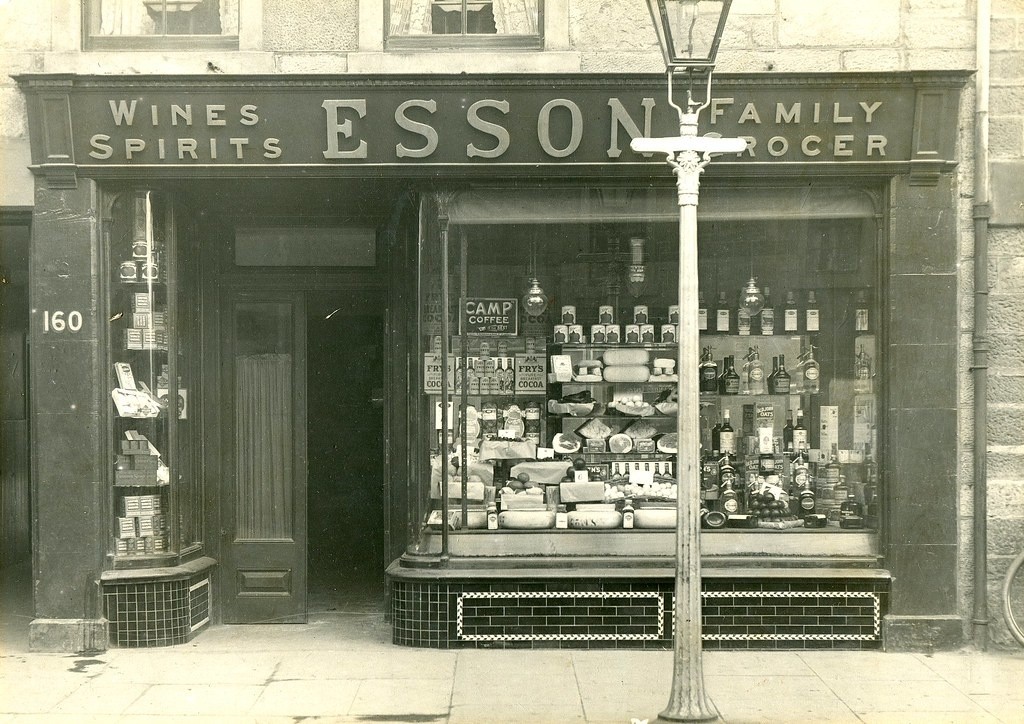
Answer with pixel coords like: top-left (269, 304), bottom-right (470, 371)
top-left (515, 354), bottom-right (547, 395)
top-left (500, 480), bottom-right (543, 511)
top-left (424, 353), bottom-right (455, 394)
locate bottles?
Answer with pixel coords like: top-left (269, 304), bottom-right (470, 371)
top-left (830, 443), bottom-right (877, 517)
top-left (854, 291), bottom-right (869, 330)
top-left (622, 499), bottom-right (634, 529)
top-left (854, 344), bottom-right (870, 391)
top-left (457, 358), bottom-right (514, 394)
top-left (487, 502), bottom-right (498, 530)
top-left (782, 409), bottom-right (815, 514)
top-left (555, 504), bottom-right (567, 529)
top-left (698, 289), bottom-right (820, 394)
top-left (613, 463), bottom-right (671, 479)
top-left (713, 409), bottom-right (738, 515)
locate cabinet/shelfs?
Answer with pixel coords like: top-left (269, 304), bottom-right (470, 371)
top-left (539, 341), bottom-right (678, 463)
top-left (430, 335), bottom-right (539, 457)
top-left (700, 335), bottom-right (813, 455)
top-left (108, 188), bottom-right (199, 567)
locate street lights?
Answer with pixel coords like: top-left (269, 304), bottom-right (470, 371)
top-left (627, 0), bottom-right (748, 722)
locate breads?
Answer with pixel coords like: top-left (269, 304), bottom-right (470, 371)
top-left (432, 457), bottom-right (681, 530)
top-left (579, 349), bottom-right (678, 442)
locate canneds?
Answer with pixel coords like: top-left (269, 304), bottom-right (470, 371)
top-left (498, 340), bottom-right (507, 357)
top-left (120, 238), bottom-right (159, 283)
top-left (468, 340), bottom-right (500, 395)
top-left (553, 304), bottom-right (679, 343)
top-left (526, 337), bottom-right (536, 353)
top-left (433, 336), bottom-right (442, 353)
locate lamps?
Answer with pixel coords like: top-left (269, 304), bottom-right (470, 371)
top-left (737, 253), bottom-right (765, 317)
top-left (522, 241), bottom-right (549, 316)
